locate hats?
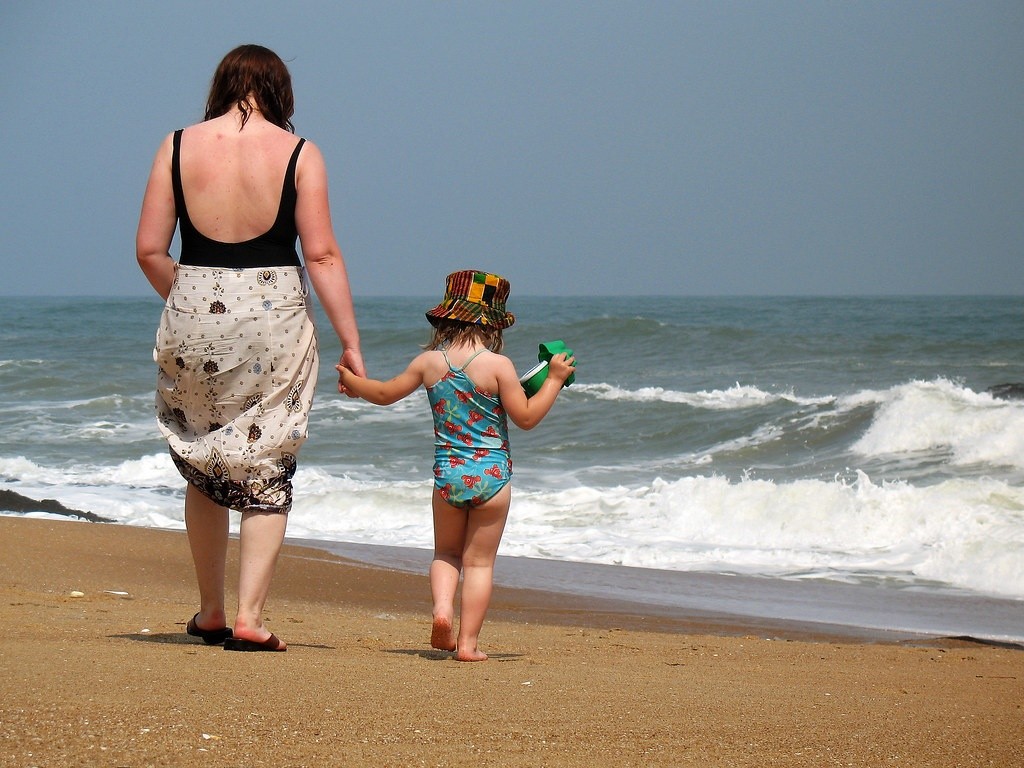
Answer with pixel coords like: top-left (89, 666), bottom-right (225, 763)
top-left (425, 270), bottom-right (514, 329)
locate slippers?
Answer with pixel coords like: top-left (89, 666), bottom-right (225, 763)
top-left (186, 611), bottom-right (233, 645)
top-left (224, 633), bottom-right (287, 651)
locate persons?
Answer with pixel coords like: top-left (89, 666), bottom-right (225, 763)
top-left (335, 270), bottom-right (576, 662)
top-left (136, 44), bottom-right (367, 651)
